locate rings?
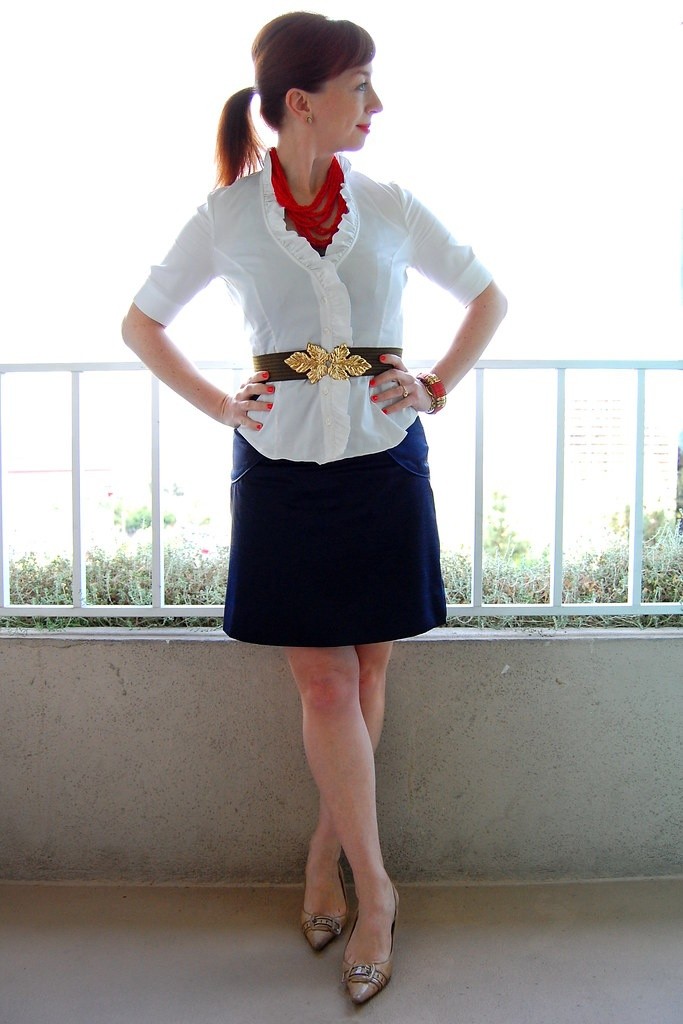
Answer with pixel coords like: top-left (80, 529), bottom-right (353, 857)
top-left (400, 386), bottom-right (409, 399)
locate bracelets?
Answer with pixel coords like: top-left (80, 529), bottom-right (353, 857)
top-left (416, 371), bottom-right (447, 416)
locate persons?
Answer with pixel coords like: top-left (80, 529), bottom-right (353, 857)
top-left (118, 9), bottom-right (516, 1012)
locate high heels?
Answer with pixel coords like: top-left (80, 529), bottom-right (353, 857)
top-left (300, 848), bottom-right (349, 950)
top-left (340, 882), bottom-right (399, 1004)
